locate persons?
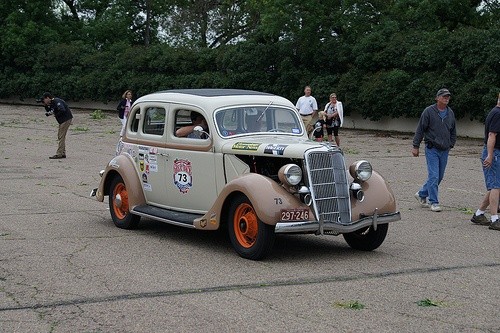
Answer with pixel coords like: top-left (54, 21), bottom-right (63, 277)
top-left (324, 93), bottom-right (343, 146)
top-left (410, 88), bottom-right (456, 211)
top-left (116, 90), bottom-right (133, 137)
top-left (41, 93), bottom-right (74, 158)
top-left (244, 110), bottom-right (264, 131)
top-left (296, 85), bottom-right (318, 139)
top-left (314, 111), bottom-right (324, 142)
top-left (469, 93), bottom-right (500, 231)
top-left (176, 111), bottom-right (206, 140)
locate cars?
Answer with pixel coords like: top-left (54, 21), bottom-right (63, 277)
top-left (90, 89), bottom-right (401, 258)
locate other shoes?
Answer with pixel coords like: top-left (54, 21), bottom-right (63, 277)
top-left (429, 203), bottom-right (441, 211)
top-left (415, 191), bottom-right (429, 208)
top-left (49, 155), bottom-right (62, 159)
top-left (62, 154), bottom-right (66, 158)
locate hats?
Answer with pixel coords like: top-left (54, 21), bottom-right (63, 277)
top-left (437, 88), bottom-right (451, 97)
top-left (40, 93), bottom-right (51, 98)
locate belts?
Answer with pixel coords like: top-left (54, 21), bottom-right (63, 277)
top-left (300, 114), bottom-right (312, 117)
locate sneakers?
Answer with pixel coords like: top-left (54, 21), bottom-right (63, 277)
top-left (489, 219), bottom-right (500, 230)
top-left (471, 213), bottom-right (490, 226)
